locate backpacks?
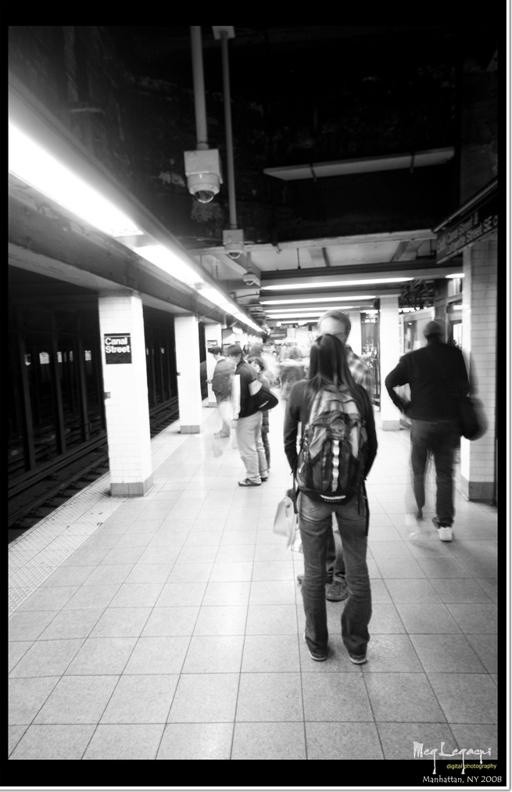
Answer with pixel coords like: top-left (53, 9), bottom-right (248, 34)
top-left (295, 380), bottom-right (369, 505)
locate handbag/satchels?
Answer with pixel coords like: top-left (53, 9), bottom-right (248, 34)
top-left (272, 488), bottom-right (301, 555)
top-left (249, 379), bottom-right (278, 411)
top-left (458, 397), bottom-right (488, 442)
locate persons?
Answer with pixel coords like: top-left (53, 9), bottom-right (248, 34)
top-left (294, 309), bottom-right (370, 603)
top-left (384, 319), bottom-right (472, 544)
top-left (346, 341), bottom-right (381, 406)
top-left (204, 337), bottom-right (310, 487)
top-left (283, 332), bottom-right (378, 664)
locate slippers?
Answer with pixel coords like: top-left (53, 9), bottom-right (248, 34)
top-left (237, 476), bottom-right (261, 487)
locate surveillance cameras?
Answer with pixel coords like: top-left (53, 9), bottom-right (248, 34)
top-left (243, 273), bottom-right (256, 285)
top-left (187, 173), bottom-right (220, 203)
top-left (224, 242), bottom-right (243, 259)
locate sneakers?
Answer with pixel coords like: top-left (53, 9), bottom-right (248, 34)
top-left (327, 580), bottom-right (348, 602)
top-left (437, 526), bottom-right (453, 542)
